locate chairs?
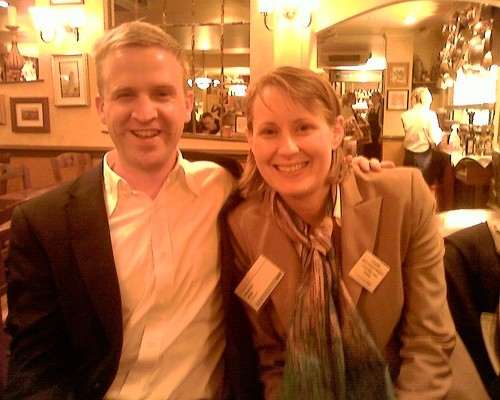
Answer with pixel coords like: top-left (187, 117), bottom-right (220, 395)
top-left (0, 196), bottom-right (31, 296)
top-left (50, 152), bottom-right (94, 181)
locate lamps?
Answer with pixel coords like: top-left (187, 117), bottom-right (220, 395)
top-left (257, 0), bottom-right (314, 32)
top-left (29, 6), bottom-right (83, 44)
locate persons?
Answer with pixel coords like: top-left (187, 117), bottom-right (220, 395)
top-left (196, 112), bottom-right (220, 134)
top-left (443, 155), bottom-right (500, 400)
top-left (227, 66), bottom-right (456, 400)
top-left (401, 87), bottom-right (446, 175)
top-left (341, 92), bottom-right (383, 162)
top-left (0, 22), bottom-right (397, 400)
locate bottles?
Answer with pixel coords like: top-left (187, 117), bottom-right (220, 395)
top-left (354, 88), bottom-right (380, 98)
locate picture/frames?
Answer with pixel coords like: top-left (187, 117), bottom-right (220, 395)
top-left (386, 89), bottom-right (409, 110)
top-left (51, 52), bottom-right (90, 108)
top-left (8, 96), bottom-right (50, 134)
top-left (387, 61), bottom-right (409, 89)
